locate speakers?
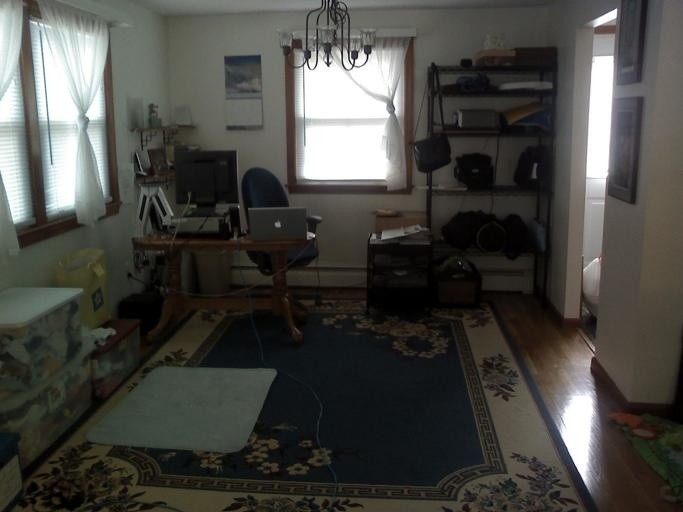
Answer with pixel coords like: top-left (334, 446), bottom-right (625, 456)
top-left (230, 206), bottom-right (240, 240)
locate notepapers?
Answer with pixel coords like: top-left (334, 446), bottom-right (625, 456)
top-left (92, 262), bottom-right (106, 277)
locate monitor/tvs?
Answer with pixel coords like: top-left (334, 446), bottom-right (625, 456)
top-left (173, 150), bottom-right (240, 217)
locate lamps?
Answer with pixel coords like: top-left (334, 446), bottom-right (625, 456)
top-left (272, 0), bottom-right (378, 72)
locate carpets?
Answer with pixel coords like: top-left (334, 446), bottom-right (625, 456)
top-left (85, 363), bottom-right (277, 454)
top-left (624, 413), bottom-right (682, 505)
top-left (5, 284), bottom-right (598, 510)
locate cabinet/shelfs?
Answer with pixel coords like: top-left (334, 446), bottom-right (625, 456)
top-left (366, 226), bottom-right (429, 321)
top-left (426, 59), bottom-right (553, 299)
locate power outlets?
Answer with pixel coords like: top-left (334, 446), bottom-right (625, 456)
top-left (121, 259), bottom-right (135, 284)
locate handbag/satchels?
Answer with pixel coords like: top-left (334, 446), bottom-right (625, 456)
top-left (410, 65), bottom-right (452, 174)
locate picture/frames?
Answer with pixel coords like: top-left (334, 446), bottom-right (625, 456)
top-left (618, 0), bottom-right (647, 87)
top-left (608, 96), bottom-right (643, 203)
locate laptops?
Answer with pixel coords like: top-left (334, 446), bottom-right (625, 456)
top-left (248, 207), bottom-right (307, 242)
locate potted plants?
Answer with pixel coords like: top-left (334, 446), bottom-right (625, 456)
top-left (435, 252), bottom-right (474, 279)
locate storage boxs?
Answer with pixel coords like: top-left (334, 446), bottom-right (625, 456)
top-left (373, 211), bottom-right (426, 233)
top-left (437, 281), bottom-right (477, 307)
top-left (0, 285), bottom-right (85, 385)
top-left (1, 353), bottom-right (92, 468)
top-left (458, 108), bottom-right (496, 130)
top-left (518, 48), bottom-right (557, 68)
top-left (91, 318), bottom-right (142, 400)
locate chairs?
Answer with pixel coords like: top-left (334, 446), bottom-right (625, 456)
top-left (244, 170), bottom-right (322, 312)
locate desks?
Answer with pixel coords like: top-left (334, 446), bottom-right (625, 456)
top-left (132, 232), bottom-right (308, 345)
